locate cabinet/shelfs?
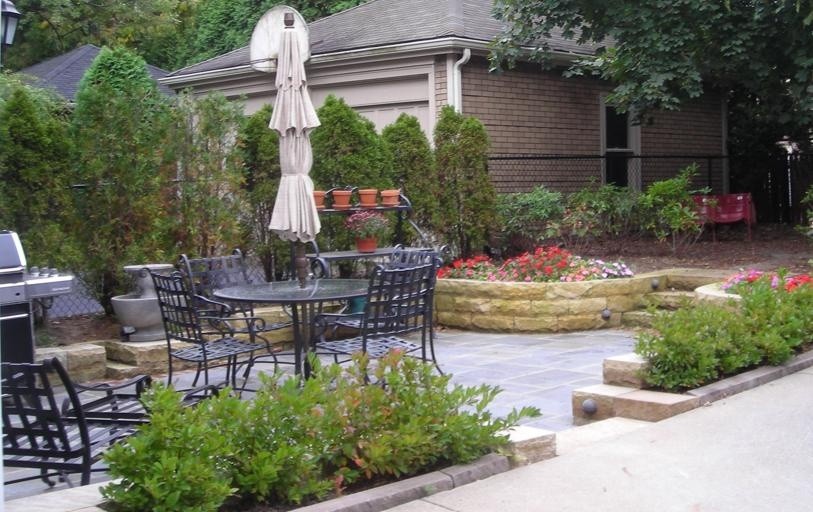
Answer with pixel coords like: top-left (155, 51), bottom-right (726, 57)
top-left (289, 185), bottom-right (444, 341)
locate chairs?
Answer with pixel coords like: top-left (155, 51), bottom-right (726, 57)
top-left (1, 357), bottom-right (223, 486)
top-left (139, 243), bottom-right (447, 399)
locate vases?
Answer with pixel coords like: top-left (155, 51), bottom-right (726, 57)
top-left (352, 235), bottom-right (379, 253)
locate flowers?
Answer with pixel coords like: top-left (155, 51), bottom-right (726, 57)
top-left (346, 210), bottom-right (390, 239)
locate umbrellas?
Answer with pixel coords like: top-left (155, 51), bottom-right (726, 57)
top-left (264, 10), bottom-right (326, 382)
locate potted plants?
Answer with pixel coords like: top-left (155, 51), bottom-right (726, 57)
top-left (380, 189), bottom-right (401, 206)
top-left (313, 189), bottom-right (327, 209)
top-left (358, 188), bottom-right (378, 208)
top-left (331, 189), bottom-right (353, 210)
